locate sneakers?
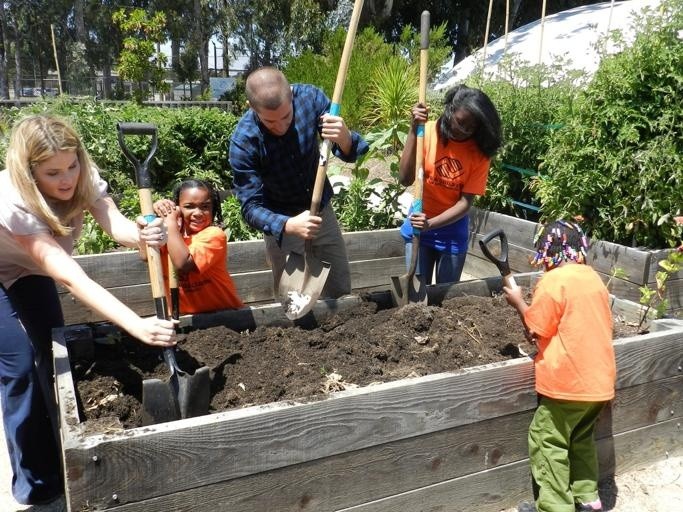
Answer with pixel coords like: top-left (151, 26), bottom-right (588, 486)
top-left (518, 500), bottom-right (538, 512)
top-left (576, 496), bottom-right (602, 511)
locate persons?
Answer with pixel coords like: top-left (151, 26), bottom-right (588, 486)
top-left (499, 211), bottom-right (617, 511)
top-left (150, 177), bottom-right (251, 314)
top-left (228, 64), bottom-right (371, 307)
top-left (0, 112), bottom-right (182, 510)
top-left (391, 82), bottom-right (503, 288)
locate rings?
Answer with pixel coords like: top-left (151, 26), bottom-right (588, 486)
top-left (159, 234), bottom-right (165, 240)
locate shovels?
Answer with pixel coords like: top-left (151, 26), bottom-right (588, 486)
top-left (392, 10), bottom-right (430, 308)
top-left (117, 122), bottom-right (211, 427)
top-left (278, 0), bottom-right (364, 322)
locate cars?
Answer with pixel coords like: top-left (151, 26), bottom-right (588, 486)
top-left (20, 88), bottom-right (46, 97)
